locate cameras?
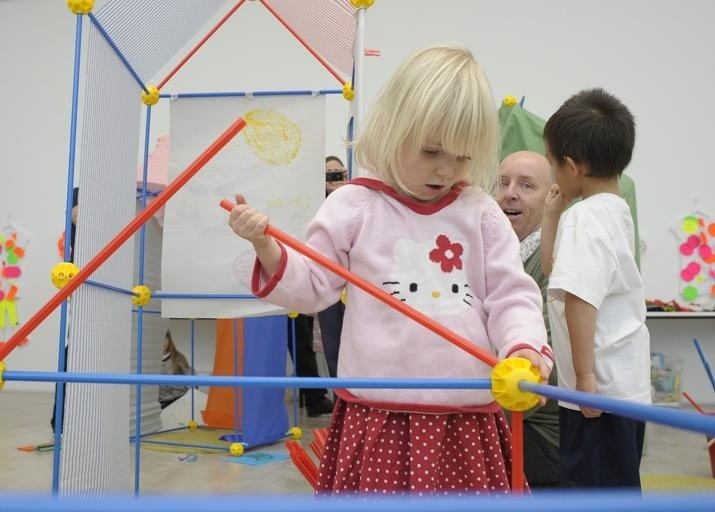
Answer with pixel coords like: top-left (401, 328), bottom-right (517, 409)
top-left (326, 172), bottom-right (344, 181)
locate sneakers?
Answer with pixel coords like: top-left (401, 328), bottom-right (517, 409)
top-left (306, 397), bottom-right (334, 418)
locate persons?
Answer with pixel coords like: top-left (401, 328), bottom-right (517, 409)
top-left (159, 329), bottom-right (199, 409)
top-left (494, 149), bottom-right (572, 489)
top-left (50, 188), bottom-right (77, 436)
top-left (228, 43), bottom-right (554, 498)
top-left (543, 87), bottom-right (652, 496)
top-left (288, 313), bottom-right (336, 417)
top-left (316, 155), bottom-right (351, 401)
